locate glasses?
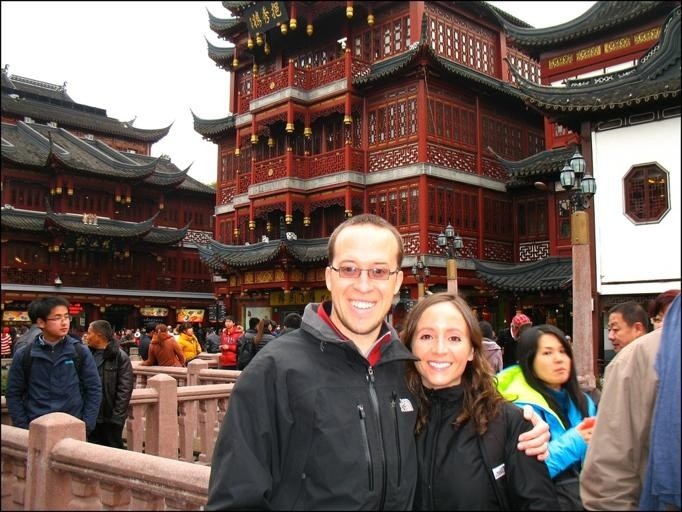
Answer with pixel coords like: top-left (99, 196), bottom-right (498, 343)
top-left (45, 315), bottom-right (73, 323)
top-left (330, 264), bottom-right (398, 282)
top-left (651, 317), bottom-right (664, 325)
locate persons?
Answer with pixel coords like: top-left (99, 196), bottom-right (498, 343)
top-left (1, 297), bottom-right (301, 449)
top-left (203, 212), bottom-right (550, 512)
top-left (399, 293), bottom-right (562, 510)
top-left (475, 287), bottom-right (680, 512)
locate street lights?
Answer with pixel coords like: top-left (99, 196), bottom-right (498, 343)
top-left (411, 259), bottom-right (430, 303)
top-left (435, 222), bottom-right (466, 297)
top-left (560, 146), bottom-right (596, 394)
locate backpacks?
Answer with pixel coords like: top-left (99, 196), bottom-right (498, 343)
top-left (237, 333), bottom-right (257, 364)
